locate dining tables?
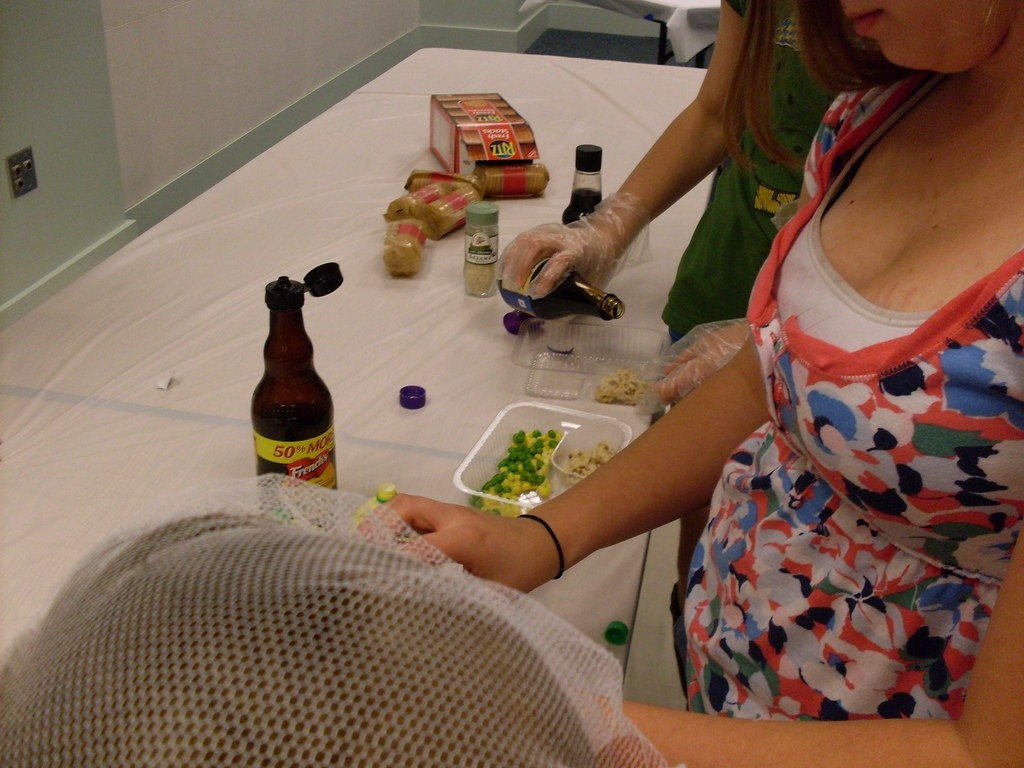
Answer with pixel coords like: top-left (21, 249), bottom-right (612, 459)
top-left (1, 46), bottom-right (712, 710)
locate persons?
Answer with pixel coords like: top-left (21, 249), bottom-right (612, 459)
top-left (353, 0), bottom-right (1024, 768)
top-left (2, 510), bottom-right (669, 768)
top-left (492, 0), bottom-right (937, 417)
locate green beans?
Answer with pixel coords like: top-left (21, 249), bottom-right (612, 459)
top-left (471, 427), bottom-right (561, 515)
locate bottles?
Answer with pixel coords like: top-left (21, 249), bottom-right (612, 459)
top-left (253, 262), bottom-right (344, 532)
top-left (351, 482), bottom-right (399, 536)
top-left (562, 145), bottom-right (603, 229)
top-left (497, 257), bottom-right (625, 320)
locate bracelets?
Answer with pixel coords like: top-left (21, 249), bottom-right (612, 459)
top-left (519, 508), bottom-right (567, 588)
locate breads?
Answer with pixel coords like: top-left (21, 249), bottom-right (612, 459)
top-left (385, 166), bottom-right (549, 274)
